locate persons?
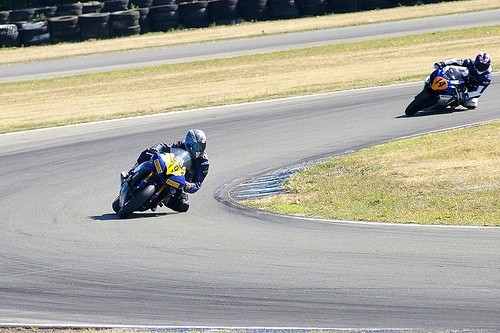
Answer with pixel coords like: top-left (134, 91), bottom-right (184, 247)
top-left (136, 128), bottom-right (211, 212)
top-left (434, 52), bottom-right (493, 108)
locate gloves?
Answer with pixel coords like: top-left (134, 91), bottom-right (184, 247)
top-left (185, 180), bottom-right (189, 191)
top-left (151, 147), bottom-right (160, 154)
top-left (434, 61), bottom-right (444, 69)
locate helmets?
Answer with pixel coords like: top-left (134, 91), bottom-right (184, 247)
top-left (475, 52), bottom-right (492, 75)
top-left (185, 130), bottom-right (206, 158)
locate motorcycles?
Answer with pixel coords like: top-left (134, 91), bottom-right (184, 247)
top-left (404, 65), bottom-right (468, 117)
top-left (112, 148), bottom-right (192, 219)
